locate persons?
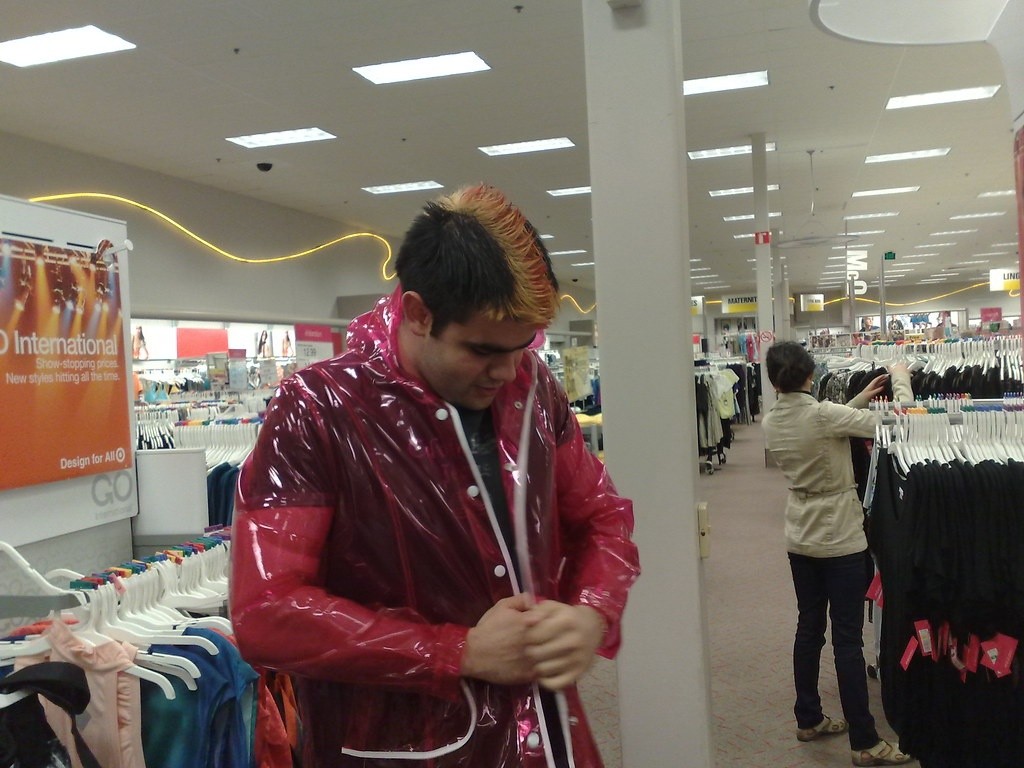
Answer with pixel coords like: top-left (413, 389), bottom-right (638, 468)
top-left (859, 315), bottom-right (879, 332)
top-left (761, 340), bottom-right (914, 768)
top-left (908, 312), bottom-right (932, 329)
top-left (258, 330), bottom-right (270, 357)
top-left (936, 311), bottom-right (956, 328)
top-left (888, 314), bottom-right (904, 331)
top-left (282, 330), bottom-right (293, 356)
top-left (229, 181), bottom-right (641, 768)
top-left (131, 325), bottom-right (149, 360)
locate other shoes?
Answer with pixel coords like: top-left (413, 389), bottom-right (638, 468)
top-left (850, 737), bottom-right (911, 767)
top-left (796, 712), bottom-right (849, 741)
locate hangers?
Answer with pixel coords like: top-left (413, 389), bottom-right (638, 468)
top-left (0, 524), bottom-right (234, 711)
top-left (133, 357), bottom-right (273, 476)
top-left (694, 355), bottom-right (741, 376)
top-left (813, 333), bottom-right (1023, 481)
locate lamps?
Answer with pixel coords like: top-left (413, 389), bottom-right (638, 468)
top-left (0, 241), bottom-right (119, 316)
